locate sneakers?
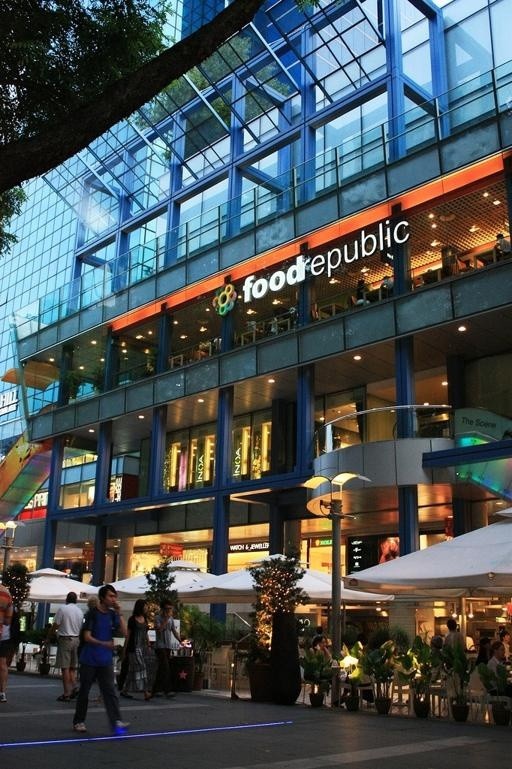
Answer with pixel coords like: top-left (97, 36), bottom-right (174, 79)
top-left (113, 720), bottom-right (130, 729)
top-left (74, 723), bottom-right (87, 731)
top-left (1, 689), bottom-right (6, 703)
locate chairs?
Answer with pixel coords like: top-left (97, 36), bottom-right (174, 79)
top-left (352, 296), bottom-right (360, 312)
top-left (312, 303), bottom-right (318, 323)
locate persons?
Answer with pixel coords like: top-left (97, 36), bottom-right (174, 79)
top-left (303, 618), bottom-right (512, 705)
top-left (357, 276), bottom-right (395, 306)
top-left (0, 583), bottom-right (185, 733)
top-left (494, 234), bottom-right (511, 260)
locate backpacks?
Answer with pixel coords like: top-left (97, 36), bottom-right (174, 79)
top-left (77, 609), bottom-right (115, 657)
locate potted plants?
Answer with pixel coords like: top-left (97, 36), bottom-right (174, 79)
top-left (296, 638), bottom-right (512, 727)
top-left (177, 600), bottom-right (224, 692)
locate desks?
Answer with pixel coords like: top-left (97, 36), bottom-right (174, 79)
top-left (171, 354), bottom-right (187, 369)
top-left (198, 341), bottom-right (212, 359)
top-left (420, 267), bottom-right (441, 286)
top-left (364, 287), bottom-right (386, 305)
top-left (241, 330), bottom-right (265, 346)
top-left (275, 318), bottom-right (293, 335)
top-left (318, 303), bottom-right (344, 321)
top-left (472, 246), bottom-right (498, 268)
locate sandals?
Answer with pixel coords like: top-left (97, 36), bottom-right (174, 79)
top-left (120, 691), bottom-right (156, 702)
top-left (56, 687), bottom-right (79, 702)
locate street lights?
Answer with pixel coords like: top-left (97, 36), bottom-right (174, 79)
top-left (300, 471), bottom-right (373, 710)
